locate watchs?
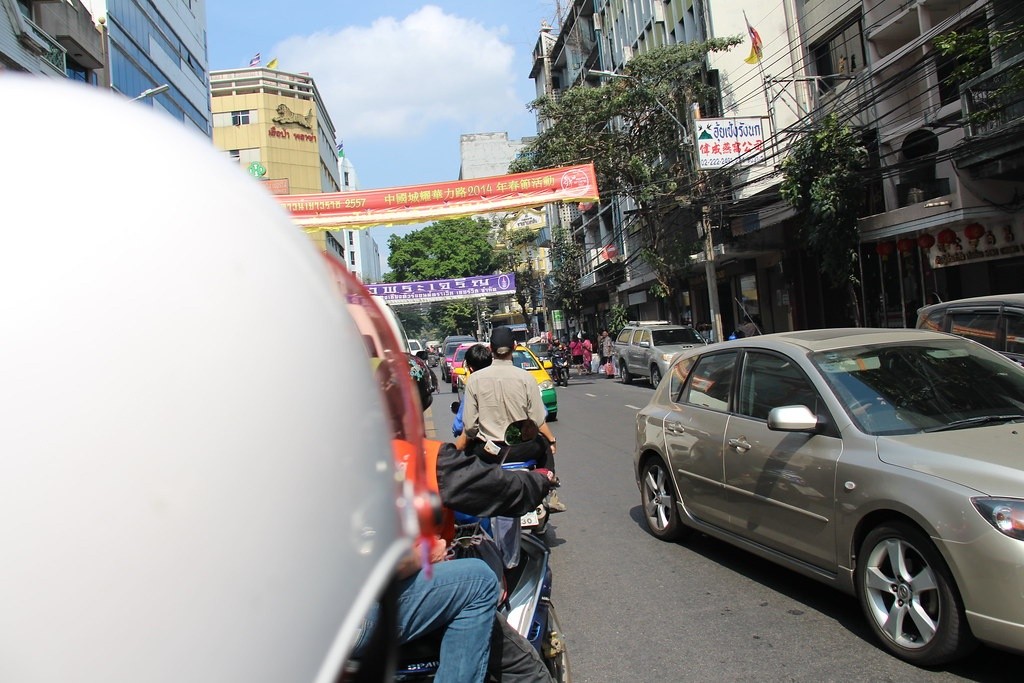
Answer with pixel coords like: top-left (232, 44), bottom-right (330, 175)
top-left (549, 437), bottom-right (556, 444)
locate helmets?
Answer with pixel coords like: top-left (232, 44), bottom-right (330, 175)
top-left (375, 353), bottom-right (439, 421)
top-left (514, 420), bottom-right (524, 432)
top-left (552, 335), bottom-right (558, 343)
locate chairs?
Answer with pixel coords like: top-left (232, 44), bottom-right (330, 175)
top-left (779, 370), bottom-right (821, 416)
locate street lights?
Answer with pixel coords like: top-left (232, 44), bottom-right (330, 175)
top-left (588, 67), bottom-right (725, 344)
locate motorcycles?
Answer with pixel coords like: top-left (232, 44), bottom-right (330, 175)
top-left (385, 414), bottom-right (574, 683)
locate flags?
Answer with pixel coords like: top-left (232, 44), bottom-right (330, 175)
top-left (250, 51), bottom-right (260, 67)
top-left (744, 18), bottom-right (763, 64)
top-left (267, 57), bottom-right (278, 68)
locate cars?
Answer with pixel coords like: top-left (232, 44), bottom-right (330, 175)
top-left (526, 342), bottom-right (556, 368)
top-left (427, 334), bottom-right (477, 382)
top-left (455, 345), bottom-right (558, 424)
top-left (409, 339), bottom-right (429, 367)
top-left (917, 295), bottom-right (1024, 368)
top-left (445, 342), bottom-right (491, 393)
top-left (639, 326), bottom-right (1024, 667)
top-left (611, 320), bottom-right (705, 392)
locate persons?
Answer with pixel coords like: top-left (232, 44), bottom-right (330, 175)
top-left (0, 73), bottom-right (567, 683)
top-left (548, 331), bottom-right (615, 379)
top-left (735, 315), bottom-right (758, 338)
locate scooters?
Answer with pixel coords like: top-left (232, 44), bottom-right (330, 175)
top-left (548, 349), bottom-right (570, 388)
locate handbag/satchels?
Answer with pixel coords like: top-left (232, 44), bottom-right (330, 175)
top-left (598, 365), bottom-right (605, 374)
top-left (604, 358), bottom-right (614, 375)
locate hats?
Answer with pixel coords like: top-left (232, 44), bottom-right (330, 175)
top-left (491, 327), bottom-right (514, 357)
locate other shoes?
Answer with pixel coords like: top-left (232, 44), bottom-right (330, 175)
top-left (543, 490), bottom-right (566, 513)
